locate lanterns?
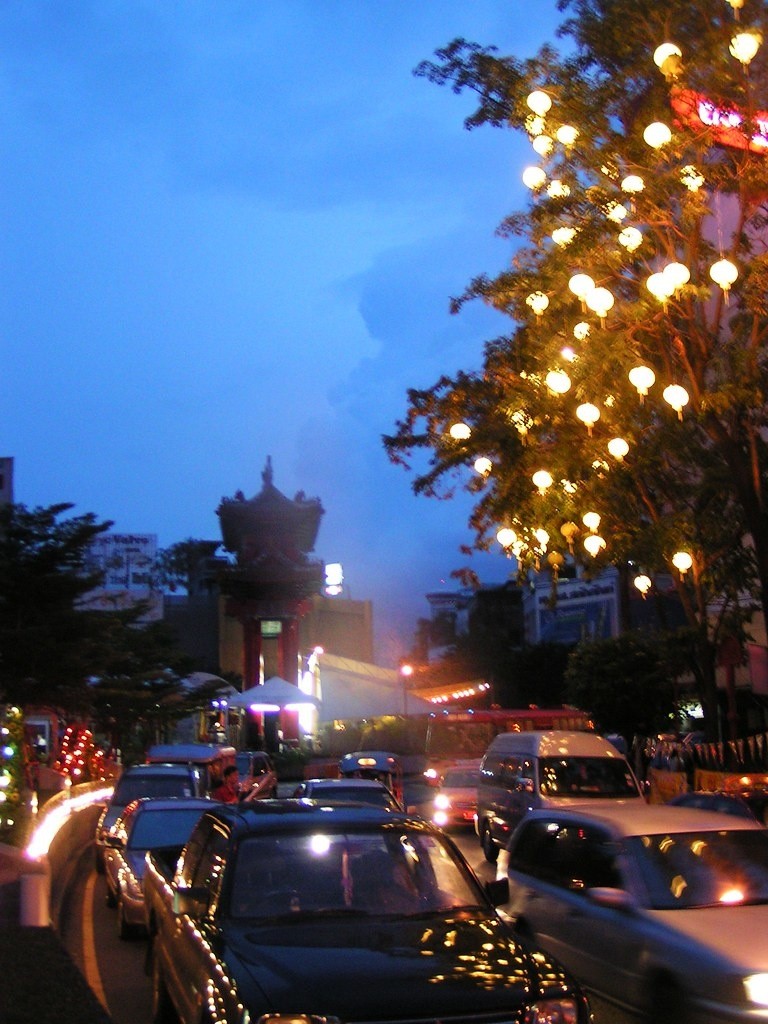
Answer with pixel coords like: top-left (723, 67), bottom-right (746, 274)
top-left (450, 30), bottom-right (759, 595)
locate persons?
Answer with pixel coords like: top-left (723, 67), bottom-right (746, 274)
top-left (213, 764), bottom-right (278, 804)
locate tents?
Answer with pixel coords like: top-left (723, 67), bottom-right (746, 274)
top-left (225, 676), bottom-right (324, 746)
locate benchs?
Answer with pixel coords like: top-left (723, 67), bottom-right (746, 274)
top-left (237, 842), bottom-right (367, 895)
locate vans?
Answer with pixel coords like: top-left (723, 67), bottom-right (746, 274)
top-left (145, 744), bottom-right (237, 793)
top-left (476, 731), bottom-right (652, 863)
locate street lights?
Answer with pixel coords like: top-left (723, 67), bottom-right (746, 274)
top-left (400, 662), bottom-right (414, 715)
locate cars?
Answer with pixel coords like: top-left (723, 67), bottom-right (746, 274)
top-left (100, 795), bottom-right (230, 942)
top-left (666, 790), bottom-right (768, 829)
top-left (292, 777), bottom-right (418, 861)
top-left (433, 765), bottom-right (481, 833)
top-left (495, 799), bottom-right (768, 1024)
top-left (337, 750), bottom-right (405, 806)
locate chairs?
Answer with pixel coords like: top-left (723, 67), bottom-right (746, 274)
top-left (341, 845), bottom-right (417, 915)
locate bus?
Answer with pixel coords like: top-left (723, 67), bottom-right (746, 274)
top-left (423, 708), bottom-right (595, 789)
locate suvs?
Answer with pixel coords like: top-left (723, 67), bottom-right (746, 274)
top-left (93, 762), bottom-right (212, 868)
top-left (226, 751), bottom-right (278, 802)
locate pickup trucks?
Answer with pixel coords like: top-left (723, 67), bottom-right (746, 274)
top-left (142, 798), bottom-right (595, 1024)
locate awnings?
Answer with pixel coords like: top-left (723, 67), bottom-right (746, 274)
top-left (319, 665), bottom-right (459, 723)
